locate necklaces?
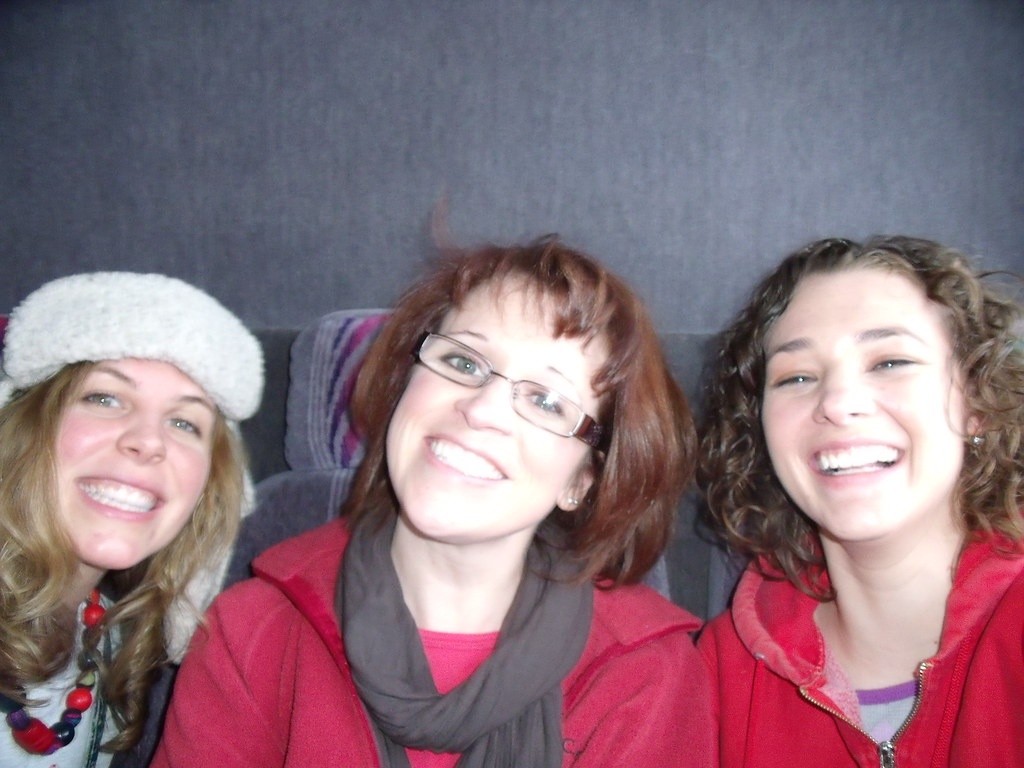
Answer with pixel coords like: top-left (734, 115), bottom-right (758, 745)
top-left (0, 590), bottom-right (105, 756)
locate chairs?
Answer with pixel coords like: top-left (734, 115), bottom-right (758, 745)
top-left (109, 313), bottom-right (672, 768)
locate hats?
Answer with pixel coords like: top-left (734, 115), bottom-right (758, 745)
top-left (0, 270), bottom-right (267, 423)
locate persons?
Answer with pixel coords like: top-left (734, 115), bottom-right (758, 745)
top-left (696, 234), bottom-right (1024, 768)
top-left (146, 241), bottom-right (714, 768)
top-left (0, 271), bottom-right (264, 768)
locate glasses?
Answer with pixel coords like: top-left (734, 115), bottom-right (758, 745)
top-left (412, 330), bottom-right (611, 451)
